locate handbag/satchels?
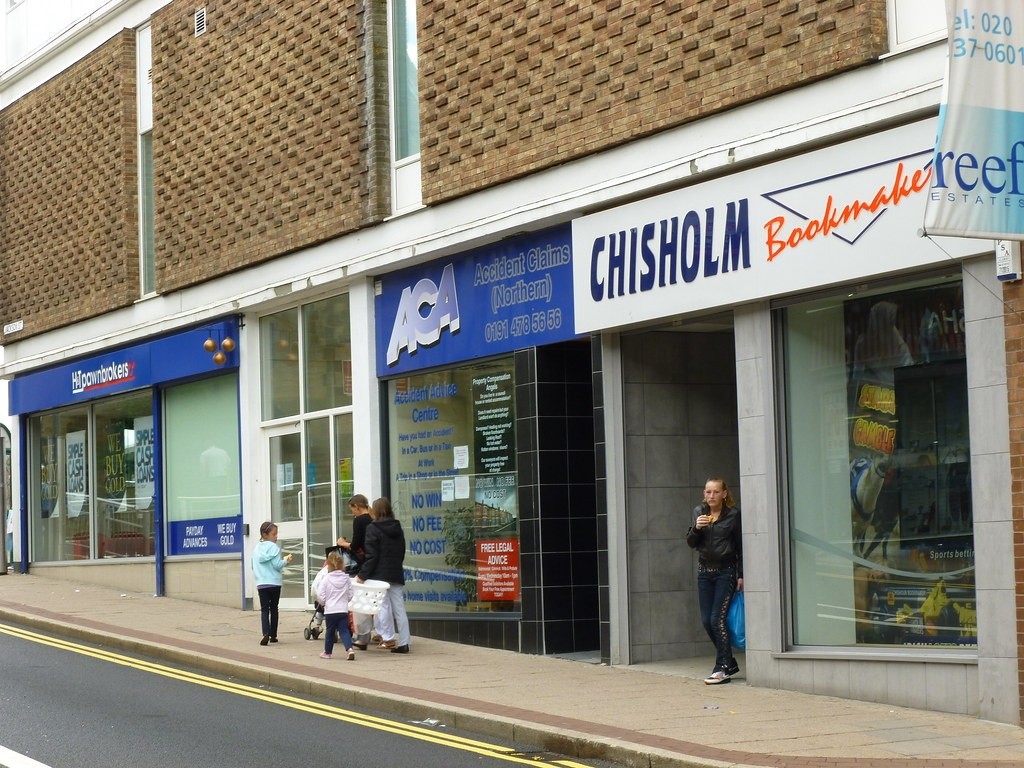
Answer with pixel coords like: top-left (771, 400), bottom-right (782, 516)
top-left (727, 588), bottom-right (746, 650)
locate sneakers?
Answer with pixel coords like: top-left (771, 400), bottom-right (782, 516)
top-left (705, 664), bottom-right (740, 685)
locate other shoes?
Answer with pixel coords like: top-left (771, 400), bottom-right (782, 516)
top-left (319, 652), bottom-right (332, 659)
top-left (270, 638), bottom-right (278, 642)
top-left (369, 636), bottom-right (383, 643)
top-left (391, 645), bottom-right (409, 653)
top-left (376, 641), bottom-right (395, 649)
top-left (352, 642), bottom-right (367, 650)
top-left (347, 652), bottom-right (355, 661)
top-left (260, 634), bottom-right (269, 646)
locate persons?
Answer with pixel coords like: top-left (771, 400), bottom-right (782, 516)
top-left (316, 493), bottom-right (411, 661)
top-left (687, 477), bottom-right (742, 685)
top-left (250, 522), bottom-right (293, 646)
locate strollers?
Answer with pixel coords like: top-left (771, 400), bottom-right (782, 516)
top-left (303, 536), bottom-right (353, 640)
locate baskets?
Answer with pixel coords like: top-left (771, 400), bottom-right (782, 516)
top-left (348, 578), bottom-right (390, 614)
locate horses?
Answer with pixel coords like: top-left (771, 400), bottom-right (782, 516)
top-left (849, 451), bottom-right (900, 560)
